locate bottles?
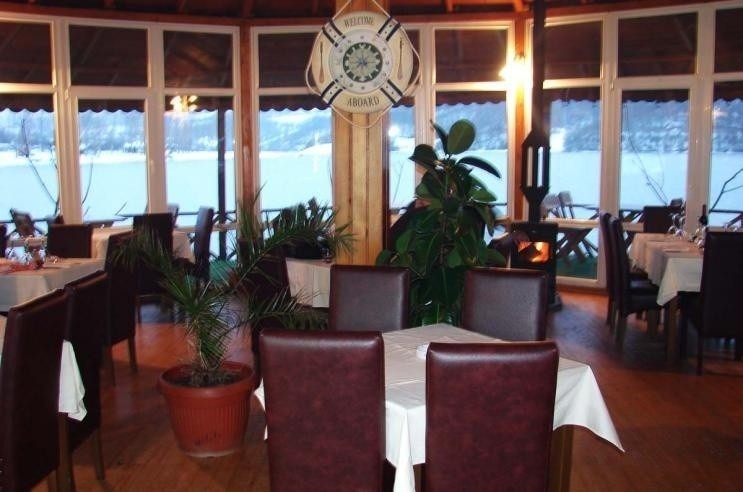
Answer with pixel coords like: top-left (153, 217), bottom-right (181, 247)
top-left (697, 204), bottom-right (708, 237)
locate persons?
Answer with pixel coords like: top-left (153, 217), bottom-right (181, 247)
top-left (385, 169), bottom-right (453, 259)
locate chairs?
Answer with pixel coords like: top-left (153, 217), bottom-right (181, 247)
top-left (461, 267), bottom-right (547, 341)
top-left (413, 341), bottom-right (559, 492)
top-left (259, 329), bottom-right (386, 491)
top-left (544, 191), bottom-right (743, 374)
top-left (63, 269), bottom-right (109, 490)
top-left (330, 264), bottom-right (410, 334)
top-left (0, 204), bottom-right (214, 322)
top-left (103, 231), bottom-right (142, 386)
top-left (1, 289), bottom-right (76, 492)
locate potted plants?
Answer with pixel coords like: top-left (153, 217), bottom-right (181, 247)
top-left (111, 181), bottom-right (361, 458)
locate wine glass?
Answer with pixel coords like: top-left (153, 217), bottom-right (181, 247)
top-left (661, 212), bottom-right (690, 252)
top-left (693, 215), bottom-right (739, 256)
top-left (1, 233), bottom-right (61, 267)
